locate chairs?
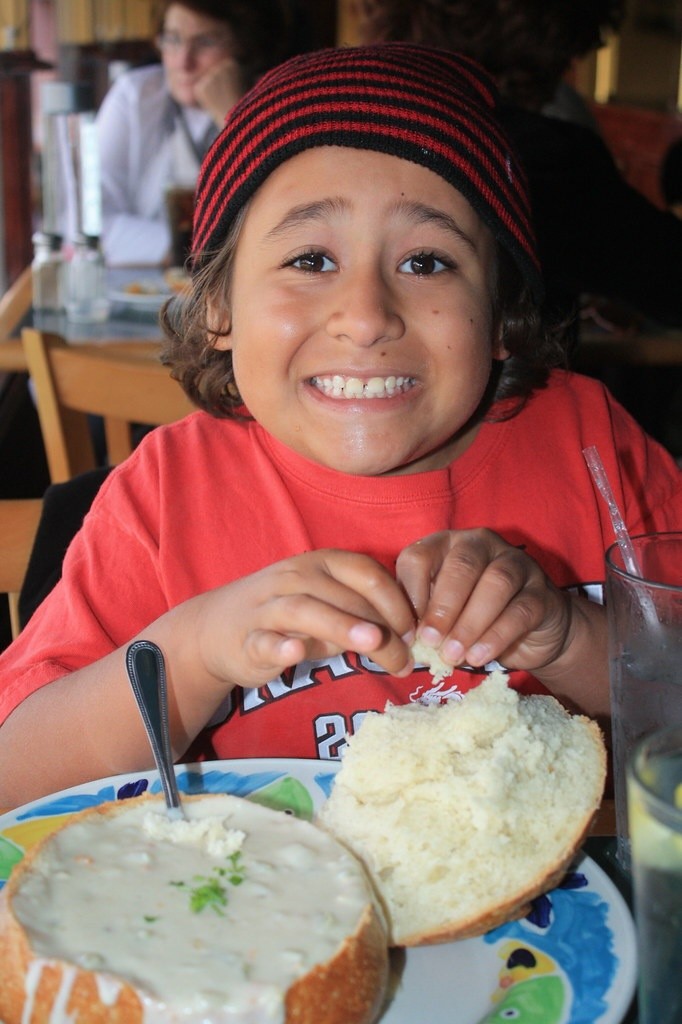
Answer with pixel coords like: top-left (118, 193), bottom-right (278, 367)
top-left (0, 496), bottom-right (45, 644)
top-left (23, 330), bottom-right (202, 478)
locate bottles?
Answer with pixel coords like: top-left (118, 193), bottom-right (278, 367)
top-left (65, 237), bottom-right (108, 323)
top-left (30, 232), bottom-right (64, 312)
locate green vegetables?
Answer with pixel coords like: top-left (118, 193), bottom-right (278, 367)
top-left (140, 853), bottom-right (246, 922)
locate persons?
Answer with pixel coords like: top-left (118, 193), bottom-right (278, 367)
top-left (0, 44), bottom-right (681, 799)
top-left (0, 0), bottom-right (682, 500)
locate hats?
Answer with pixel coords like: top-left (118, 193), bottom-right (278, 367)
top-left (191, 41), bottom-right (547, 314)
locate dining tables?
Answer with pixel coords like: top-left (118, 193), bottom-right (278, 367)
top-left (0, 265), bottom-right (171, 371)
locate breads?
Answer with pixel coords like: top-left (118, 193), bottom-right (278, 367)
top-left (0, 672), bottom-right (609, 1024)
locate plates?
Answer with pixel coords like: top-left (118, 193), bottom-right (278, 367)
top-left (109, 290), bottom-right (170, 312)
top-left (0, 758), bottom-right (636, 1024)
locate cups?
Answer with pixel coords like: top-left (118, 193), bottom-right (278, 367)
top-left (605, 531), bottom-right (682, 1024)
top-left (165, 186), bottom-right (194, 267)
